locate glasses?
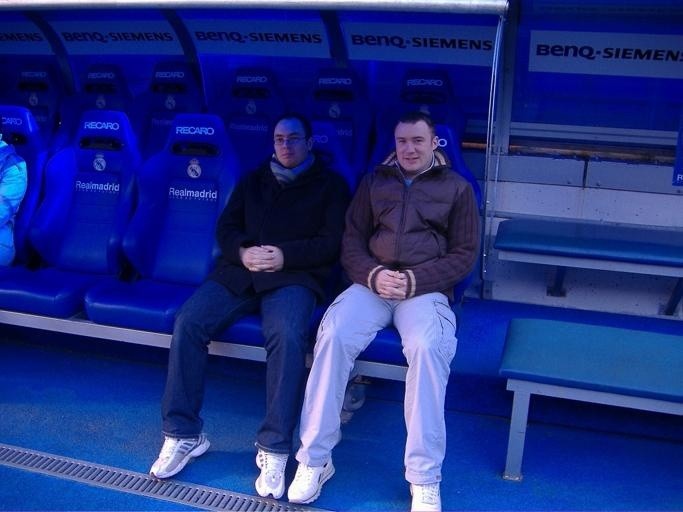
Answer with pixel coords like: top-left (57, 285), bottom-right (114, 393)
top-left (272, 137), bottom-right (299, 145)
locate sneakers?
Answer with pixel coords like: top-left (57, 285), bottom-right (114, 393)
top-left (287, 461), bottom-right (335, 505)
top-left (150, 433), bottom-right (211, 480)
top-left (410, 481), bottom-right (442, 512)
top-left (254, 447), bottom-right (289, 500)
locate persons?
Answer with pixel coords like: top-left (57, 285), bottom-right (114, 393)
top-left (148, 110), bottom-right (351, 499)
top-left (287, 110), bottom-right (481, 512)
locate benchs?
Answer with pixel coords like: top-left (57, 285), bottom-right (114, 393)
top-left (492, 217), bottom-right (683, 317)
top-left (497, 316), bottom-right (683, 484)
